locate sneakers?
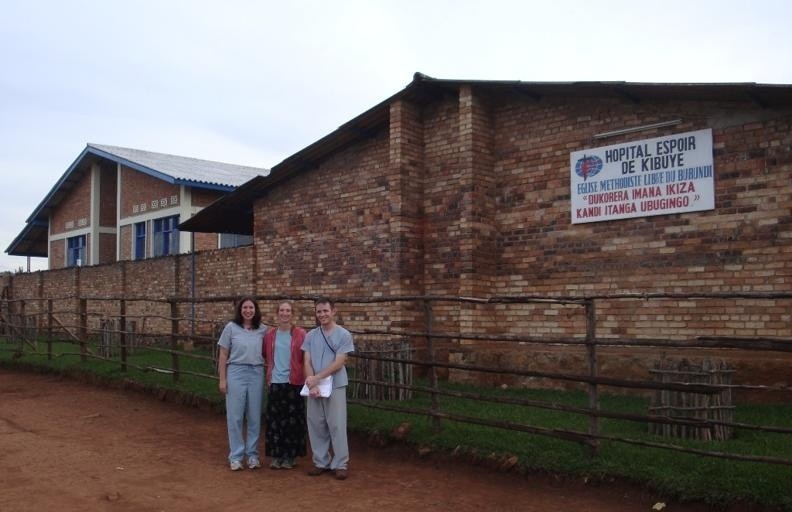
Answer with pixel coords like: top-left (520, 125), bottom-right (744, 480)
top-left (231, 455), bottom-right (261, 470)
top-left (307, 467), bottom-right (328, 476)
top-left (270, 458), bottom-right (295, 469)
top-left (335, 470), bottom-right (348, 479)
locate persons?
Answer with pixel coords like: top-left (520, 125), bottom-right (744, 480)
top-left (218, 296), bottom-right (355, 479)
